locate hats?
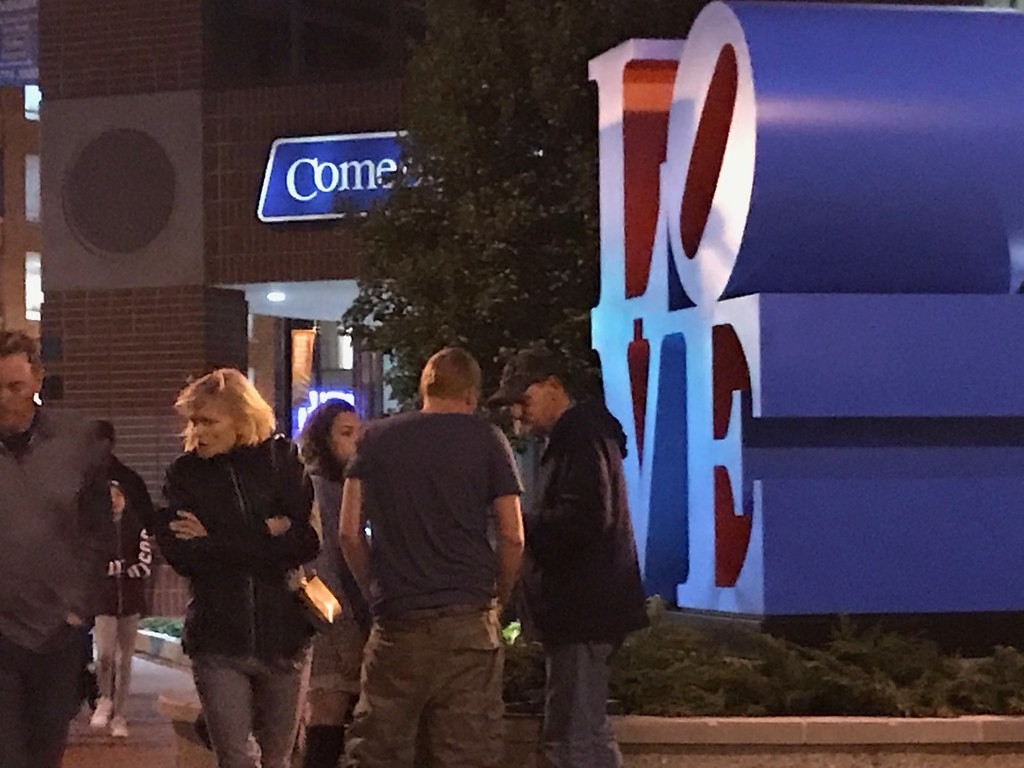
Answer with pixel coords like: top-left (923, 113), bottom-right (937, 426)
top-left (109, 480), bottom-right (127, 498)
top-left (486, 346), bottom-right (565, 408)
top-left (86, 419), bottom-right (115, 441)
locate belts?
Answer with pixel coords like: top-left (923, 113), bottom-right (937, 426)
top-left (395, 603), bottom-right (473, 622)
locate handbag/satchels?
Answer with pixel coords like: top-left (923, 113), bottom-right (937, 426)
top-left (297, 575), bottom-right (342, 634)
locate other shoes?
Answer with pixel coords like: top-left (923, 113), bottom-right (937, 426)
top-left (111, 715), bottom-right (128, 737)
top-left (90, 696), bottom-right (113, 728)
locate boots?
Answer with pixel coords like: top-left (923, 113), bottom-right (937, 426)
top-left (302, 724), bottom-right (346, 768)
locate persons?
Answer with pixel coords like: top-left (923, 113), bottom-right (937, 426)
top-left (77, 349), bottom-right (652, 768)
top-left (0, 331), bottom-right (112, 768)
